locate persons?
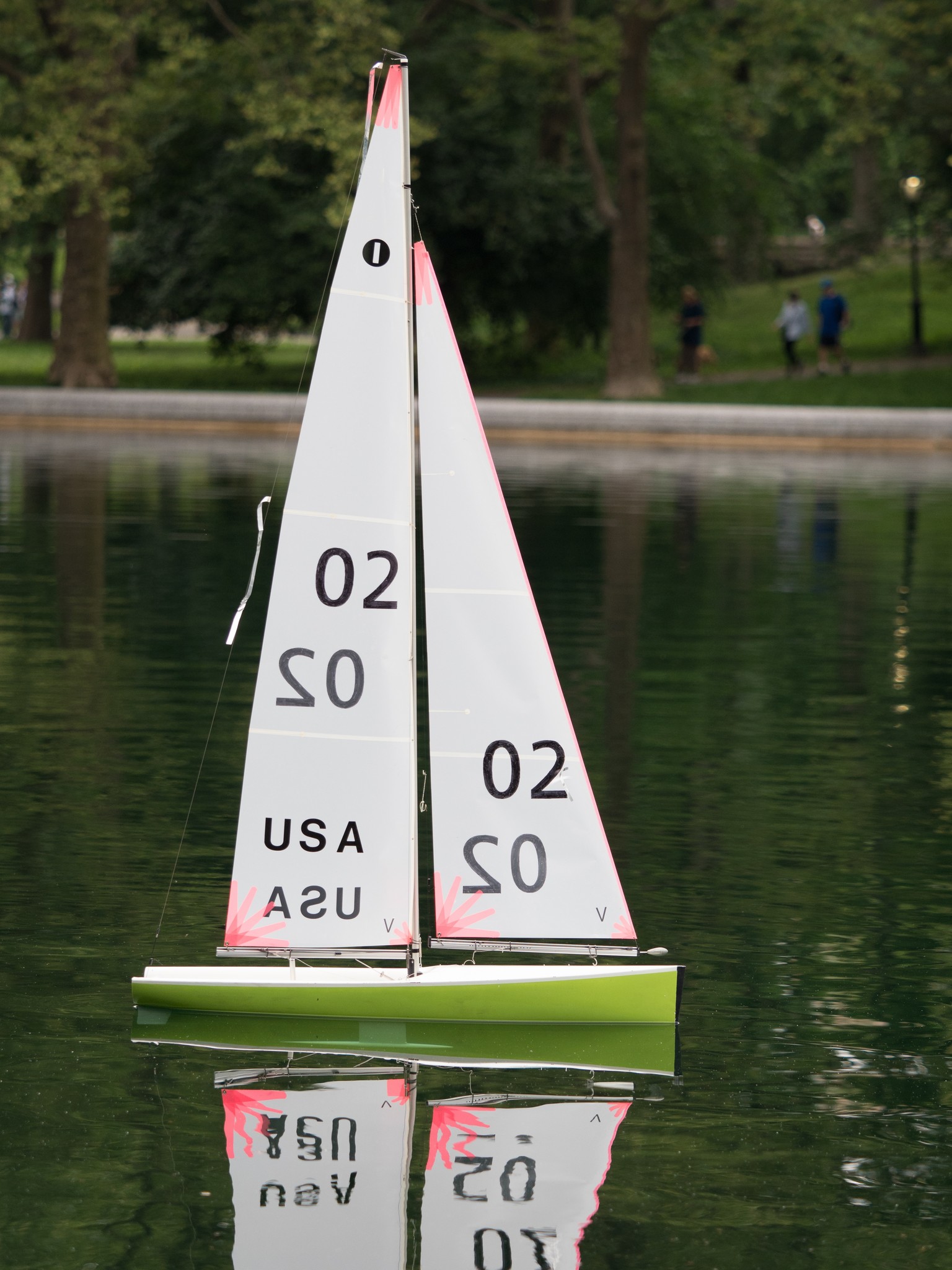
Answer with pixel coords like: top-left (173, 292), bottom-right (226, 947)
top-left (815, 280), bottom-right (852, 380)
top-left (673, 285), bottom-right (709, 373)
top-left (772, 286), bottom-right (809, 375)
top-left (0, 274), bottom-right (29, 337)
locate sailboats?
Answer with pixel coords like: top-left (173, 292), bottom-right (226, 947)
top-left (129, 1005), bottom-right (677, 1270)
top-left (129, 42), bottom-right (688, 1033)
